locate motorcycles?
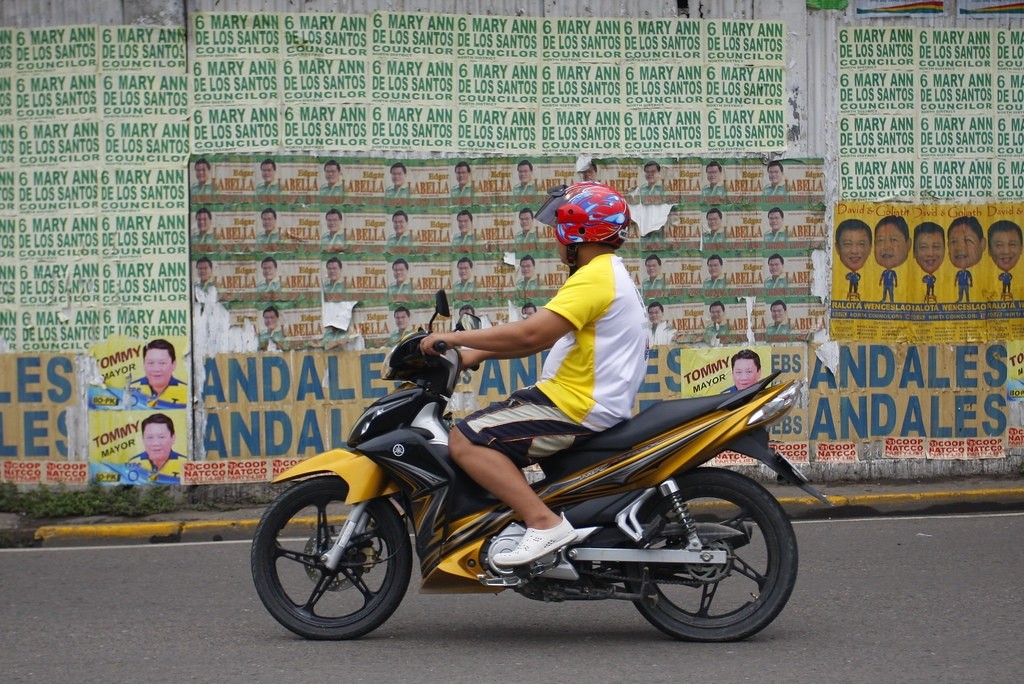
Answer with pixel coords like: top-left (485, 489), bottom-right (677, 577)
top-left (248, 291), bottom-right (841, 644)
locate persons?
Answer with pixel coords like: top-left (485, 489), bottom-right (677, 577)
top-left (835, 213), bottom-right (1024, 274)
top-left (128, 413), bottom-right (187, 477)
top-left (420, 182), bottom-right (650, 567)
top-left (128, 339), bottom-right (187, 407)
top-left (719, 349), bottom-right (763, 396)
top-left (190, 159), bottom-right (796, 335)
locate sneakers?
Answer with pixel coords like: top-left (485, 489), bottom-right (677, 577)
top-left (490, 514), bottom-right (579, 566)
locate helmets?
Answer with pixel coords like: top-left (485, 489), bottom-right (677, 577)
top-left (534, 181), bottom-right (632, 276)
